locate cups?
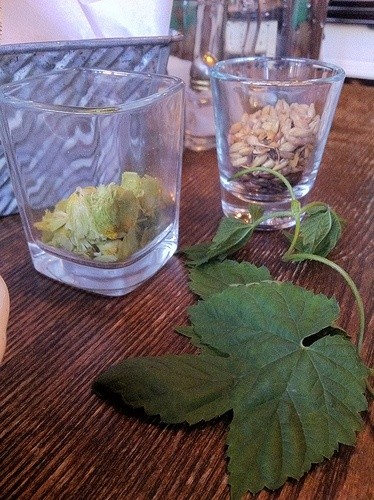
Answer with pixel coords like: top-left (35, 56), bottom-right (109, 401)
top-left (210, 56), bottom-right (345, 232)
top-left (1, 67), bottom-right (187, 297)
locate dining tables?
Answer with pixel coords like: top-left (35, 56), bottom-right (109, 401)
top-left (1, 75), bottom-right (374, 500)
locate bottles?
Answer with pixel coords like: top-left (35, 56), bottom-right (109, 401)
top-left (185, 53), bottom-right (217, 153)
